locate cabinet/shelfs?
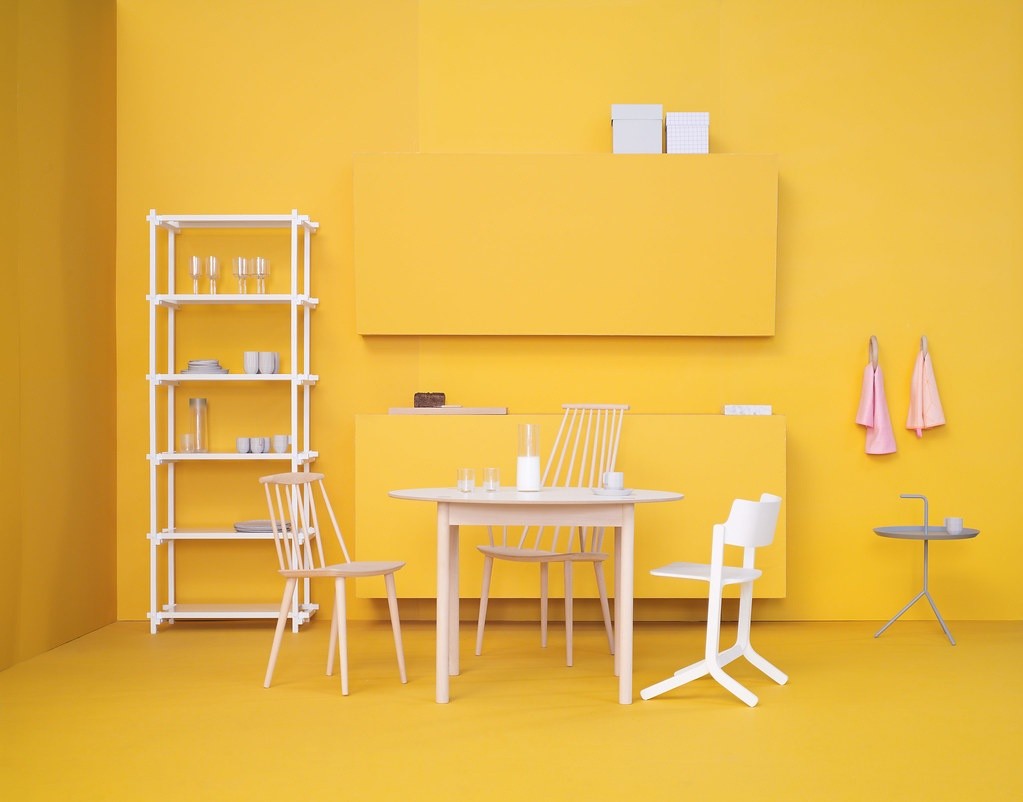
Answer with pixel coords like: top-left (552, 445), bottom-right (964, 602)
top-left (146, 208), bottom-right (318, 635)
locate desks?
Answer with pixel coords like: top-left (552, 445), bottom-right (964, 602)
top-left (387, 487), bottom-right (683, 705)
top-left (873, 495), bottom-right (980, 646)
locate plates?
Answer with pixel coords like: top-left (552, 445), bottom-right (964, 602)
top-left (592, 488), bottom-right (634, 495)
top-left (233, 519), bottom-right (291, 533)
top-left (180, 359), bottom-right (228, 374)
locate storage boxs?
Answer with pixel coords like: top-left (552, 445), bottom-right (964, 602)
top-left (665, 113), bottom-right (710, 154)
top-left (610, 104), bottom-right (662, 154)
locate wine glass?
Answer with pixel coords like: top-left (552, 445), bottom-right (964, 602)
top-left (233, 256), bottom-right (271, 295)
top-left (188, 255), bottom-right (219, 296)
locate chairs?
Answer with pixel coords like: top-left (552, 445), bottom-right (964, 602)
top-left (259, 473), bottom-right (407, 696)
top-left (475, 403), bottom-right (630, 667)
top-left (640, 493), bottom-right (789, 707)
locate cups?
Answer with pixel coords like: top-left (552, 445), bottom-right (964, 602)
top-left (236, 434), bottom-right (292, 453)
top-left (517, 422), bottom-right (541, 492)
top-left (945, 518), bottom-right (963, 535)
top-left (483, 467), bottom-right (501, 493)
top-left (602, 472), bottom-right (624, 490)
top-left (187, 398), bottom-right (209, 453)
top-left (456, 468), bottom-right (475, 494)
top-left (180, 433), bottom-right (196, 453)
top-left (243, 351), bottom-right (280, 375)
